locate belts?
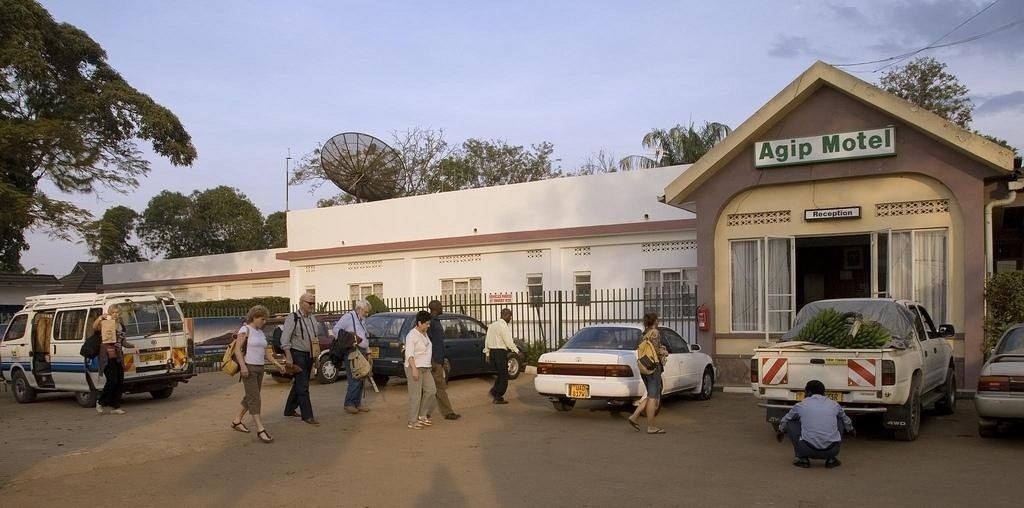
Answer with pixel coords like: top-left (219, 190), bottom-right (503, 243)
top-left (803, 439), bottom-right (834, 450)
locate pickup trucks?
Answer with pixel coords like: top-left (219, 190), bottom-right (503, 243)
top-left (750, 297), bottom-right (961, 440)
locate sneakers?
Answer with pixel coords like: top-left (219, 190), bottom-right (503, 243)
top-left (344, 405), bottom-right (358, 413)
top-left (418, 415), bottom-right (432, 425)
top-left (110, 408), bottom-right (126, 413)
top-left (490, 387), bottom-right (496, 396)
top-left (96, 399), bottom-right (104, 415)
top-left (407, 420), bottom-right (425, 429)
top-left (356, 405), bottom-right (369, 411)
top-left (445, 412), bottom-right (461, 418)
top-left (493, 399), bottom-right (508, 403)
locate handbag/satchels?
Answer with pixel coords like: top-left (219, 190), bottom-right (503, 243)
top-left (101, 319), bottom-right (116, 343)
top-left (80, 334), bottom-right (102, 358)
top-left (310, 336), bottom-right (321, 357)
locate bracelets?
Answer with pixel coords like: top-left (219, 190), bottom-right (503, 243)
top-left (273, 362), bottom-right (277, 366)
top-left (98, 317), bottom-right (103, 321)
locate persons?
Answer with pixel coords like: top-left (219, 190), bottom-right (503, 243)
top-left (628, 311), bottom-right (669, 434)
top-left (281, 293), bottom-right (319, 425)
top-left (80, 303), bottom-right (139, 414)
top-left (427, 300), bottom-right (461, 421)
top-left (231, 306), bottom-right (286, 444)
top-left (777, 379), bottom-right (855, 468)
top-left (404, 311), bottom-right (437, 429)
top-left (483, 309), bottom-right (522, 404)
top-left (333, 299), bottom-right (372, 413)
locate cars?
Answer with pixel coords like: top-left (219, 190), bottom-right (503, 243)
top-left (534, 323), bottom-right (713, 418)
top-left (977, 322), bottom-right (1024, 436)
top-left (363, 313), bottom-right (527, 386)
top-left (264, 313), bottom-right (351, 384)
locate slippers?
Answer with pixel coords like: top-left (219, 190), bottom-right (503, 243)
top-left (648, 427), bottom-right (665, 433)
top-left (628, 418), bottom-right (640, 431)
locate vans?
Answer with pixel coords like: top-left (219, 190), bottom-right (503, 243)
top-left (1, 290), bottom-right (193, 400)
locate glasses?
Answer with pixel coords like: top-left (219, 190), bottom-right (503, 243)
top-left (303, 299), bottom-right (315, 304)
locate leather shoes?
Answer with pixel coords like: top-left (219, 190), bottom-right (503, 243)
top-left (792, 457), bottom-right (809, 467)
top-left (825, 457), bottom-right (841, 467)
top-left (285, 409), bottom-right (301, 416)
top-left (303, 417), bottom-right (319, 424)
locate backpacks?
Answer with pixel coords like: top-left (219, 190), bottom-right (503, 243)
top-left (221, 324), bottom-right (249, 381)
top-left (273, 311), bottom-right (304, 353)
top-left (348, 348), bottom-right (371, 379)
top-left (637, 328), bottom-right (659, 374)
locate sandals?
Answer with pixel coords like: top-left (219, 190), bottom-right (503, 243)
top-left (231, 421), bottom-right (250, 432)
top-left (257, 428), bottom-right (273, 442)
